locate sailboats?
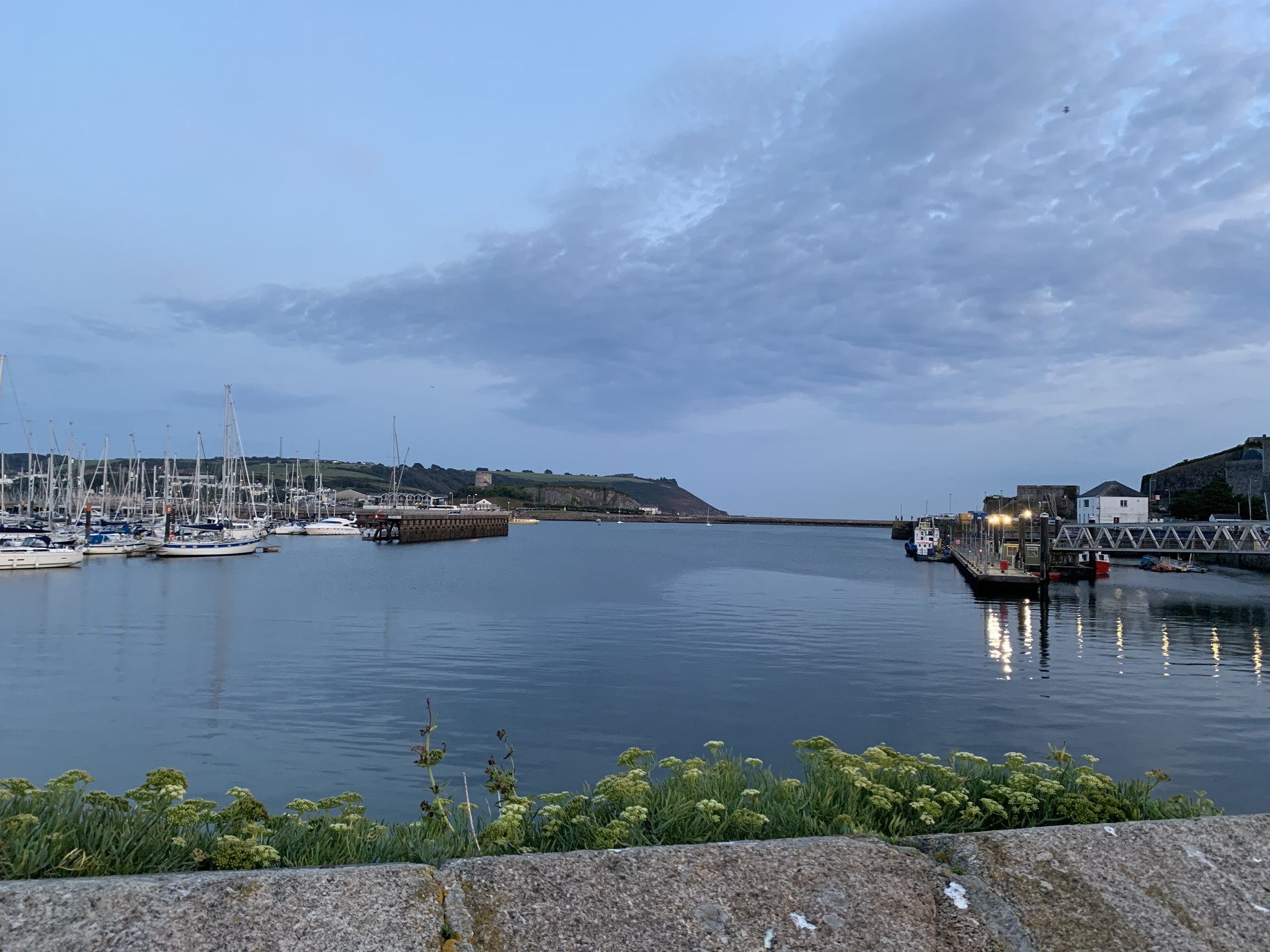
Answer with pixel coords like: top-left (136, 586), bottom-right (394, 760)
top-left (617, 504), bottom-right (623, 524)
top-left (706, 506), bottom-right (712, 527)
top-left (0, 385), bottom-right (407, 569)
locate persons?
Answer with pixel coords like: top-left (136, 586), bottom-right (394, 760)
top-left (1015, 551), bottom-right (1020, 568)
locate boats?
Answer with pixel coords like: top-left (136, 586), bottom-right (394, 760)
top-left (508, 519), bottom-right (542, 525)
top-left (1140, 555), bottom-right (1208, 576)
top-left (1001, 545), bottom-right (1111, 581)
top-left (905, 498), bottom-right (952, 565)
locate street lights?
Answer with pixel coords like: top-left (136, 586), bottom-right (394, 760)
top-left (473, 494), bottom-right (478, 511)
top-left (949, 493), bottom-right (952, 514)
top-left (1000, 490), bottom-right (1003, 514)
top-left (426, 490), bottom-right (432, 509)
top-left (1149, 479), bottom-right (1160, 507)
top-left (449, 492), bottom-right (453, 510)
top-left (984, 491), bottom-right (987, 512)
top-left (508, 502), bottom-right (510, 512)
top-left (1165, 489), bottom-right (1171, 514)
top-left (467, 495), bottom-right (471, 510)
